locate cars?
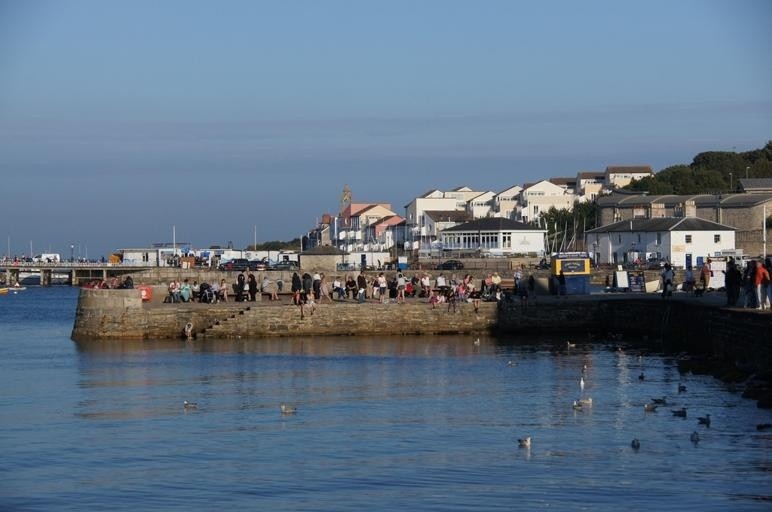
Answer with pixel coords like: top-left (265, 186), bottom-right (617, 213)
top-left (437, 260), bottom-right (463, 270)
top-left (640, 258), bottom-right (665, 270)
top-left (248, 261), bottom-right (267, 271)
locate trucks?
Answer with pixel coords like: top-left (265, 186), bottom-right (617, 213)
top-left (32, 254), bottom-right (61, 263)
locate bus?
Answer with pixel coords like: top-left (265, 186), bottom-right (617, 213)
top-left (707, 255), bottom-right (752, 269)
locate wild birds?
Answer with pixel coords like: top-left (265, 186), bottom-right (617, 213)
top-left (473, 338), bottom-right (480, 344)
top-left (280, 404), bottom-right (297, 413)
top-left (567, 341), bottom-right (712, 446)
top-left (507, 360), bottom-right (520, 366)
top-left (183, 400), bottom-right (198, 407)
top-left (517, 436), bottom-right (531, 446)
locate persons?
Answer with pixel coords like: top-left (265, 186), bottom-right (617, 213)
top-left (111, 275), bottom-right (118, 289)
top-left (659, 258), bottom-right (771, 311)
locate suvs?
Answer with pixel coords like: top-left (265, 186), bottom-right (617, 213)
top-left (219, 259), bottom-right (250, 271)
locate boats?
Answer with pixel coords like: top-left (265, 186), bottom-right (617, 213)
top-left (6, 286), bottom-right (27, 291)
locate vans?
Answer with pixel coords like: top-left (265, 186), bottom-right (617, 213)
top-left (271, 261), bottom-right (297, 270)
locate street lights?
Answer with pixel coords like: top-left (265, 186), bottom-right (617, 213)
top-left (70, 243), bottom-right (75, 260)
top-left (729, 172), bottom-right (733, 189)
top-left (746, 167), bottom-right (750, 178)
